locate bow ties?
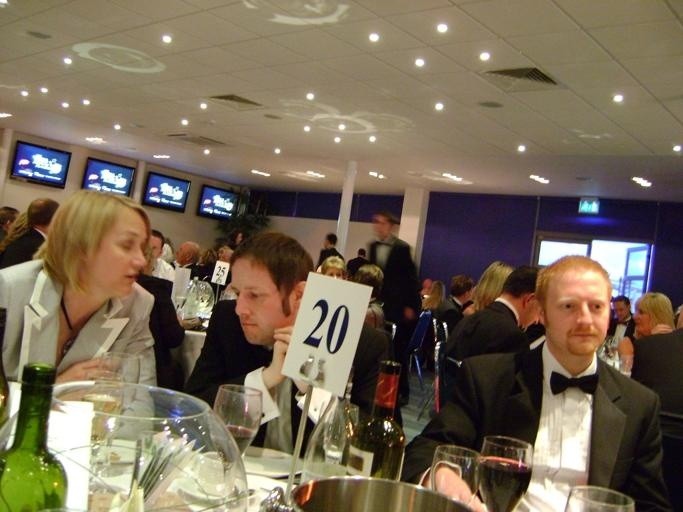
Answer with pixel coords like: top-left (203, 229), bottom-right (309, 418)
top-left (550, 371), bottom-right (598, 394)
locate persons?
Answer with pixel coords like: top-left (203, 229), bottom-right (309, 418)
top-left (0, 190), bottom-right (158, 437)
top-left (401, 255), bottom-right (672, 511)
top-left (0, 207), bottom-right (20, 241)
top-left (315, 233), bottom-right (345, 271)
top-left (437, 274), bottom-right (475, 344)
top-left (321, 256), bottom-right (347, 280)
top-left (176, 241), bottom-right (218, 301)
top-left (230, 230), bottom-right (242, 247)
top-left (444, 266), bottom-right (540, 359)
top-left (347, 249), bottom-right (372, 278)
top-left (198, 247), bottom-right (217, 278)
top-left (218, 245), bottom-right (234, 302)
top-left (354, 264), bottom-right (384, 326)
top-left (140, 229), bottom-right (177, 312)
top-left (605, 296), bottom-right (636, 348)
top-left (135, 245), bottom-right (186, 349)
top-left (421, 281), bottom-right (445, 315)
top-left (369, 209), bottom-right (422, 407)
top-left (619, 293), bottom-right (676, 377)
top-left (164, 238), bottom-right (176, 270)
top-left (460, 260), bottom-right (517, 319)
top-left (183, 233), bottom-right (379, 460)
top-left (159, 243), bottom-right (175, 264)
top-left (1, 198), bottom-right (60, 270)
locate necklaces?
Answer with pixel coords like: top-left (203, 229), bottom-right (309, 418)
top-left (60, 299), bottom-right (75, 353)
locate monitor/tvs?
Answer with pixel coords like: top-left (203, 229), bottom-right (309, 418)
top-left (142, 171), bottom-right (192, 213)
top-left (10, 140), bottom-right (73, 189)
top-left (81, 157), bottom-right (138, 199)
top-left (197, 184), bottom-right (241, 222)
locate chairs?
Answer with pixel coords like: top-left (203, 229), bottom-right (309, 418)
top-left (418, 342), bottom-right (452, 422)
top-left (437, 319), bottom-right (450, 347)
top-left (406, 309), bottom-right (433, 390)
top-left (426, 313), bottom-right (441, 342)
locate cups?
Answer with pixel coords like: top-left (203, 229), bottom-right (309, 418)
top-left (564, 485), bottom-right (634, 512)
top-left (90, 350), bottom-right (140, 460)
top-left (428, 445), bottom-right (477, 505)
top-left (80, 368), bottom-right (124, 480)
top-left (476, 433), bottom-right (532, 511)
top-left (209, 384), bottom-right (262, 495)
top-left (320, 400), bottom-right (359, 464)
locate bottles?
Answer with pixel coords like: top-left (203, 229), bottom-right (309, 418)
top-left (1, 363), bottom-right (69, 511)
top-left (345, 360), bottom-right (405, 480)
top-left (0, 308), bottom-right (11, 461)
